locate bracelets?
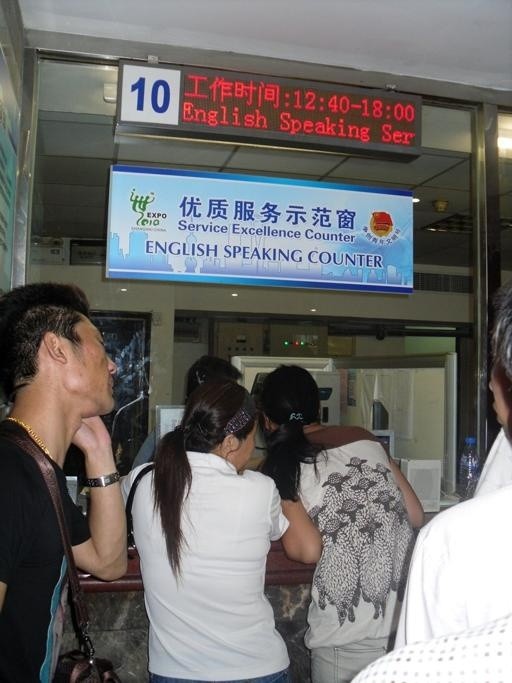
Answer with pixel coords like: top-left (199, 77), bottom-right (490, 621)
top-left (82, 469), bottom-right (122, 488)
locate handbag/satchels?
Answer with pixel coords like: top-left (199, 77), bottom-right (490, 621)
top-left (50, 649), bottom-right (122, 683)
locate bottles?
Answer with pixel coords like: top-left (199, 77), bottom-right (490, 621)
top-left (458, 437), bottom-right (481, 502)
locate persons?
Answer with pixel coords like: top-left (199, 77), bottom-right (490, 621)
top-left (130, 352), bottom-right (245, 472)
top-left (0, 279), bottom-right (131, 682)
top-left (120, 376), bottom-right (293, 682)
top-left (255, 361), bottom-right (426, 682)
top-left (394, 277), bottom-right (512, 652)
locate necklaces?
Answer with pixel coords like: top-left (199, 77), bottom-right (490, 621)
top-left (5, 414), bottom-right (54, 461)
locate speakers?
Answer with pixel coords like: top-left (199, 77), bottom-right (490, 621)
top-left (400, 458), bottom-right (442, 516)
